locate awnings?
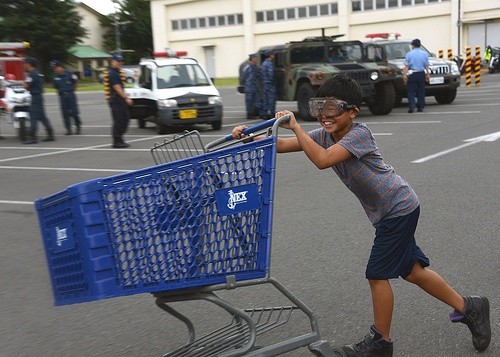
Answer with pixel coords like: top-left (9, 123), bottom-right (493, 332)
top-left (66, 45), bottom-right (113, 58)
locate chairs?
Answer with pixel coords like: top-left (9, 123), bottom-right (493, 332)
top-left (169, 76), bottom-right (182, 87)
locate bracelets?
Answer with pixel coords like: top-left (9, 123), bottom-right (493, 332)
top-left (292, 124), bottom-right (298, 129)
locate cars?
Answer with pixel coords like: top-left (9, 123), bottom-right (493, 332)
top-left (98, 66), bottom-right (138, 84)
top-left (124, 51), bottom-right (223, 135)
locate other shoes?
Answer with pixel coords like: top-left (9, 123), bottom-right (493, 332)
top-left (408, 108), bottom-right (414, 113)
top-left (42, 135), bottom-right (54, 141)
top-left (248, 113), bottom-right (260, 119)
top-left (64, 129), bottom-right (72, 135)
top-left (417, 108), bottom-right (424, 112)
top-left (264, 113), bottom-right (274, 119)
top-left (113, 140), bottom-right (130, 147)
top-left (22, 137), bottom-right (38, 144)
top-left (75, 126), bottom-right (80, 134)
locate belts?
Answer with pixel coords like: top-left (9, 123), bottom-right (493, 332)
top-left (412, 69), bottom-right (423, 72)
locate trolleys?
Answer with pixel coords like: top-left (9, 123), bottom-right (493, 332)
top-left (34, 114), bottom-right (348, 357)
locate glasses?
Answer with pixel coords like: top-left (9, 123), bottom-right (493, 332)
top-left (307, 98), bottom-right (358, 119)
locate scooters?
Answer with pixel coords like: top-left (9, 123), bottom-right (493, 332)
top-left (0, 73), bottom-right (33, 142)
top-left (486, 46), bottom-right (500, 74)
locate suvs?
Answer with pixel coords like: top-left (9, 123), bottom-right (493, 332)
top-left (345, 32), bottom-right (462, 108)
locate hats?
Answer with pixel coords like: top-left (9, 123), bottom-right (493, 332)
top-left (114, 53), bottom-right (125, 60)
top-left (22, 57), bottom-right (38, 67)
top-left (265, 50), bottom-right (277, 56)
top-left (49, 60), bottom-right (60, 69)
top-left (250, 54), bottom-right (258, 58)
top-left (410, 39), bottom-right (420, 45)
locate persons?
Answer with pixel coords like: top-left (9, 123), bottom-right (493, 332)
top-left (254, 50), bottom-right (276, 120)
top-left (328, 49), bottom-right (344, 62)
top-left (106, 53), bottom-right (134, 148)
top-left (402, 39), bottom-right (430, 113)
top-left (240, 53), bottom-right (258, 117)
top-left (50, 59), bottom-right (81, 135)
top-left (84, 63), bottom-right (92, 76)
top-left (23, 57), bottom-right (54, 144)
top-left (231, 72), bottom-right (492, 357)
top-left (340, 49), bottom-right (353, 60)
top-left (485, 46), bottom-right (493, 63)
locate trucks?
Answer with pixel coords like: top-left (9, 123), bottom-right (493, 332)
top-left (237, 28), bottom-right (402, 121)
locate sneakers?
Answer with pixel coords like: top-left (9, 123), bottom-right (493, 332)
top-left (449, 295), bottom-right (492, 353)
top-left (342, 324), bottom-right (393, 357)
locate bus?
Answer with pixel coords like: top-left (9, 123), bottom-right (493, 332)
top-left (0, 42), bottom-right (30, 108)
